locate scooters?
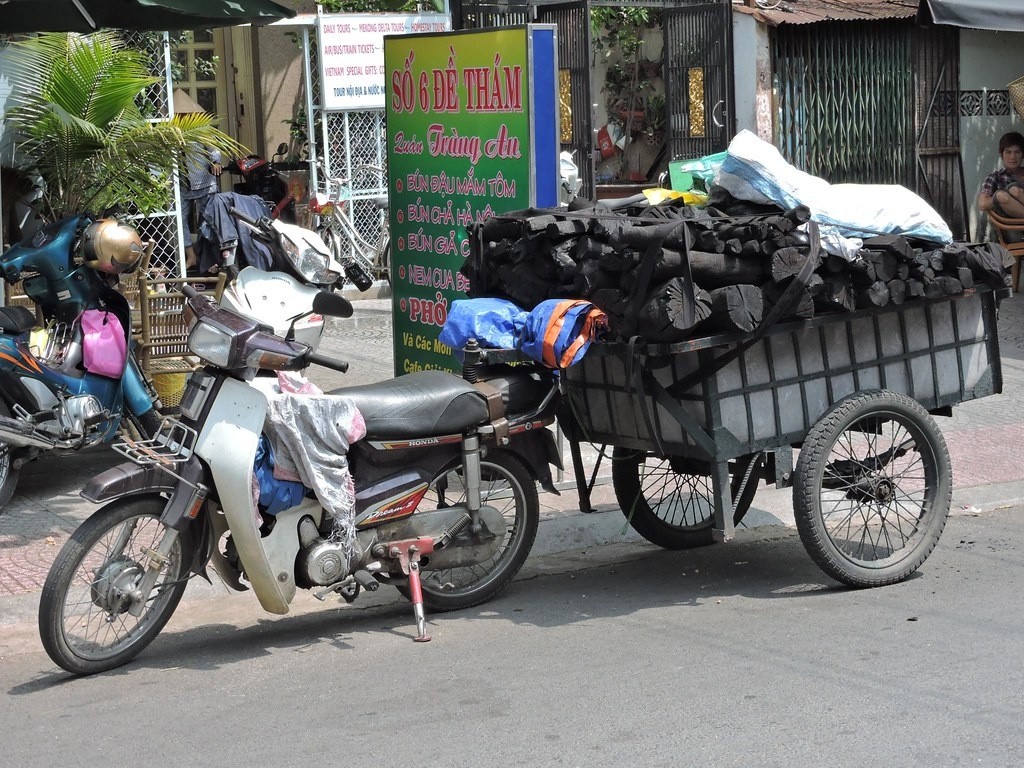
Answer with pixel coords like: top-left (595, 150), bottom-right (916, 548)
top-left (221, 143), bottom-right (296, 278)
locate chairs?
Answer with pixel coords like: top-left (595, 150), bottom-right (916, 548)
top-left (988, 210), bottom-right (1024, 293)
top-left (135, 270), bottom-right (226, 380)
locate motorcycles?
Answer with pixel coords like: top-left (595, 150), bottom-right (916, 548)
top-left (221, 206), bottom-right (370, 349)
top-left (0, 210), bottom-right (166, 509)
top-left (36, 284), bottom-right (562, 676)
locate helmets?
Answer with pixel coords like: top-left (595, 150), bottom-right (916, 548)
top-left (81, 220), bottom-right (142, 276)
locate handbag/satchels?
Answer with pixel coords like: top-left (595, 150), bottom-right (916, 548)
top-left (80, 309), bottom-right (126, 380)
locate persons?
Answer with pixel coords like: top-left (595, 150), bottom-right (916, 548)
top-left (978, 131), bottom-right (1024, 243)
top-left (177, 112), bottom-right (224, 270)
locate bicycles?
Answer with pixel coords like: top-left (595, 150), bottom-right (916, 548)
top-left (305, 155), bottom-right (394, 288)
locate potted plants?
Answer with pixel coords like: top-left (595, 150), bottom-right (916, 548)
top-left (619, 96), bottom-right (644, 130)
top-left (641, 95), bottom-right (666, 146)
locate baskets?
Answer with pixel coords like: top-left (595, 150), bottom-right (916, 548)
top-left (1007, 75), bottom-right (1024, 120)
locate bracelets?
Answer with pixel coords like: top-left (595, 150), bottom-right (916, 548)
top-left (213, 160), bottom-right (220, 164)
